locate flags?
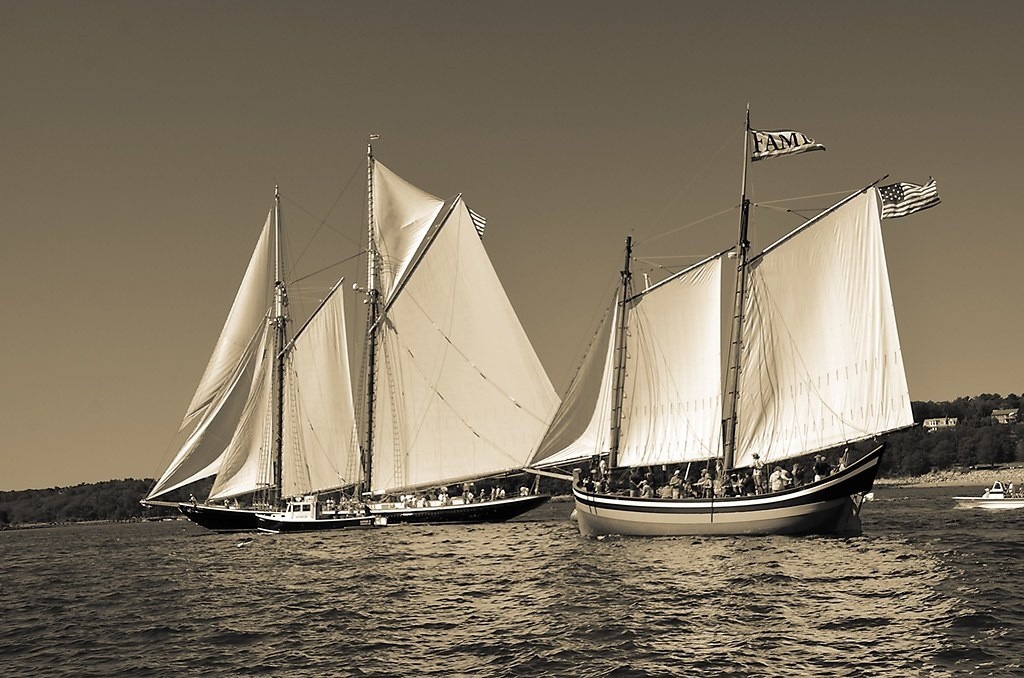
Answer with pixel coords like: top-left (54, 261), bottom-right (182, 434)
top-left (747, 126), bottom-right (826, 162)
top-left (877, 176), bottom-right (942, 221)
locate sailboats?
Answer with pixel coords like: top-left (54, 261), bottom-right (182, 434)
top-left (522, 101), bottom-right (946, 535)
top-left (140, 130), bottom-right (555, 535)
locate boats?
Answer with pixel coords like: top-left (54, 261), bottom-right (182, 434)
top-left (954, 478), bottom-right (1024, 511)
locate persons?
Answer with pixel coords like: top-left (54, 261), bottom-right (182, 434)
top-left (190, 494), bottom-right (199, 506)
top-left (326, 497), bottom-right (334, 511)
top-left (584, 447), bottom-right (850, 500)
top-left (224, 498), bottom-right (229, 509)
top-left (400, 484), bottom-right (529, 509)
top-left (1008, 480), bottom-right (1014, 498)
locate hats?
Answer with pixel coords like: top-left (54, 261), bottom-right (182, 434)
top-left (640, 480), bottom-right (647, 483)
top-left (814, 455), bottom-right (821, 458)
top-left (821, 457), bottom-right (827, 461)
top-left (751, 453), bottom-right (758, 456)
top-left (701, 469), bottom-right (707, 472)
top-left (674, 470), bottom-right (679, 475)
